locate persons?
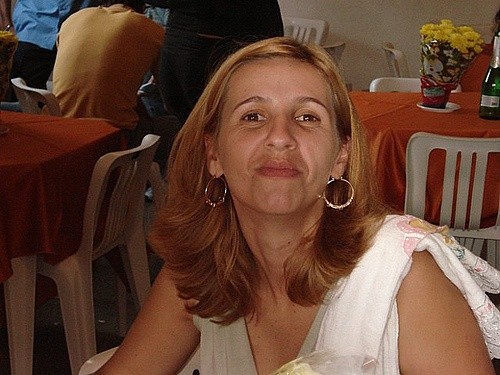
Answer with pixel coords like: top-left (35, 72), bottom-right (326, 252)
top-left (88, 38), bottom-right (500, 375)
top-left (0, 0), bottom-right (285, 201)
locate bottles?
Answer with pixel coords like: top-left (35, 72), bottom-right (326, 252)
top-left (145, 5), bottom-right (165, 27)
top-left (479, 35), bottom-right (500, 121)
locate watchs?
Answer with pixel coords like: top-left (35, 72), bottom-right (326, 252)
top-left (6, 24), bottom-right (13, 31)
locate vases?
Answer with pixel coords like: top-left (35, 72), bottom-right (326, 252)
top-left (418, 76), bottom-right (458, 108)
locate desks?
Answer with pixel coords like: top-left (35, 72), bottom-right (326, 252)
top-left (0, 108), bottom-right (128, 299)
top-left (347, 90), bottom-right (500, 230)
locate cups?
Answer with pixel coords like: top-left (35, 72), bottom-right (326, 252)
top-left (270, 353), bottom-right (381, 375)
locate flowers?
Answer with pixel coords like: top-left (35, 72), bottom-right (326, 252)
top-left (418, 18), bottom-right (484, 55)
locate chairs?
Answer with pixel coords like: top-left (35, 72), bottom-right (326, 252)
top-left (4, 16), bottom-right (500, 375)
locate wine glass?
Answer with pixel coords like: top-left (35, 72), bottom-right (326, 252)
top-left (0, 29), bottom-right (20, 133)
